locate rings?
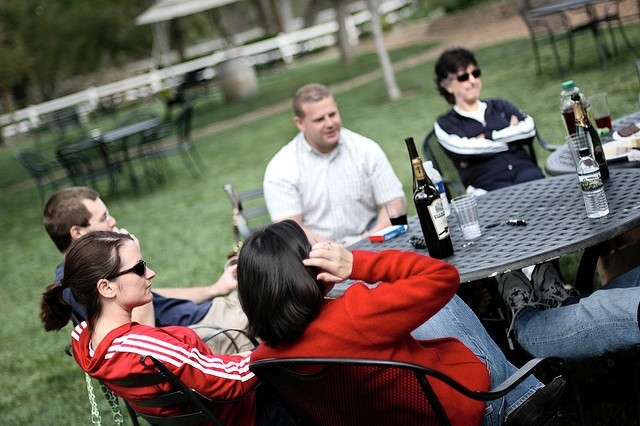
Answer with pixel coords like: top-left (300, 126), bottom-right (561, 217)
top-left (326, 241), bottom-right (332, 250)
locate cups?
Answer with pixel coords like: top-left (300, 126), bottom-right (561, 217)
top-left (588, 92), bottom-right (614, 132)
top-left (383, 198), bottom-right (408, 230)
top-left (451, 195), bottom-right (485, 240)
top-left (566, 132), bottom-right (596, 180)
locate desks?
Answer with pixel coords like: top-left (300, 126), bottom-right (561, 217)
top-left (323, 166), bottom-right (640, 368)
top-left (544, 109), bottom-right (640, 176)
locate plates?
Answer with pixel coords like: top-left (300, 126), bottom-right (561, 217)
top-left (625, 149), bottom-right (639, 161)
top-left (598, 140), bottom-right (627, 162)
top-left (612, 123), bottom-right (638, 146)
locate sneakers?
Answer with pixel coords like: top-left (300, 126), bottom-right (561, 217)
top-left (531, 263), bottom-right (580, 307)
top-left (505, 374), bottom-right (566, 424)
top-left (497, 269), bottom-right (548, 338)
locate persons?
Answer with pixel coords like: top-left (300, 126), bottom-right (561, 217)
top-left (148, 63), bottom-right (207, 183)
top-left (236, 221), bottom-right (567, 425)
top-left (433, 46), bottom-right (546, 199)
top-left (263, 82), bottom-right (407, 247)
top-left (90, 91), bottom-right (134, 190)
top-left (498, 262), bottom-right (640, 361)
top-left (41, 186), bottom-right (262, 357)
top-left (40, 230), bottom-right (297, 425)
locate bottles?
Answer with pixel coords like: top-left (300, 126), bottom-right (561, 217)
top-left (576, 147), bottom-right (610, 220)
top-left (571, 93), bottom-right (609, 182)
top-left (423, 160), bottom-right (451, 219)
top-left (558, 80), bottom-right (596, 141)
top-left (404, 137), bottom-right (453, 260)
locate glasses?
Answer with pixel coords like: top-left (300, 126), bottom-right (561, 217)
top-left (448, 70), bottom-right (480, 81)
top-left (106, 262), bottom-right (146, 280)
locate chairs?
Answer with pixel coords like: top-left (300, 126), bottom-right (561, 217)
top-left (248, 356), bottom-right (570, 426)
top-left (67, 326), bottom-right (257, 426)
top-left (422, 118), bottom-right (561, 198)
top-left (223, 180), bottom-right (272, 255)
